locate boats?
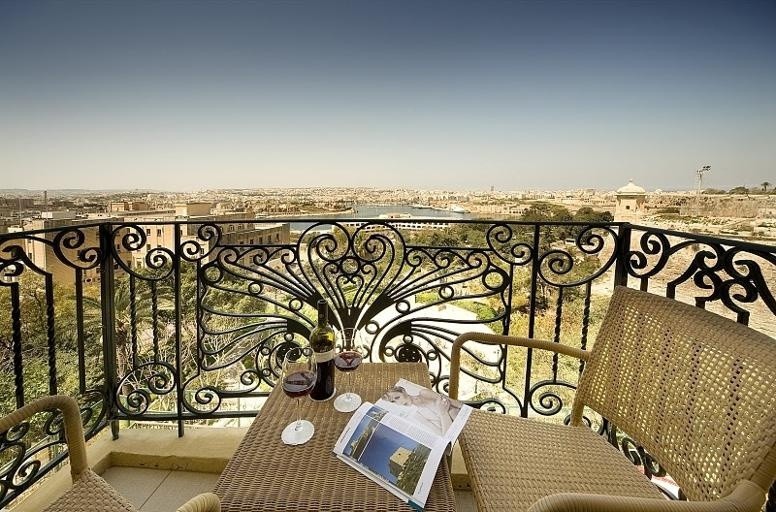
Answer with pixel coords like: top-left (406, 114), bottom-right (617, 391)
top-left (451, 206), bottom-right (465, 213)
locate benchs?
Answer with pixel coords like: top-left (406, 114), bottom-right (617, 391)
top-left (450, 284), bottom-right (775, 511)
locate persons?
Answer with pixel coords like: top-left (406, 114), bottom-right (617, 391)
top-left (382, 386), bottom-right (463, 436)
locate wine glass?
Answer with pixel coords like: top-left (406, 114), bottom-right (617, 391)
top-left (280, 347), bottom-right (317, 447)
top-left (332, 328), bottom-right (363, 414)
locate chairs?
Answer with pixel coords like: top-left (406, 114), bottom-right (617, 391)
top-left (1, 394), bottom-right (221, 512)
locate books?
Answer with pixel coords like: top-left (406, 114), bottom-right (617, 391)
top-left (333, 378), bottom-right (474, 512)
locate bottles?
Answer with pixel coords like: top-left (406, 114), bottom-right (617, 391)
top-left (307, 300), bottom-right (337, 403)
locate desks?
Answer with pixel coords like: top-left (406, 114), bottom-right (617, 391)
top-left (210, 362), bottom-right (459, 512)
